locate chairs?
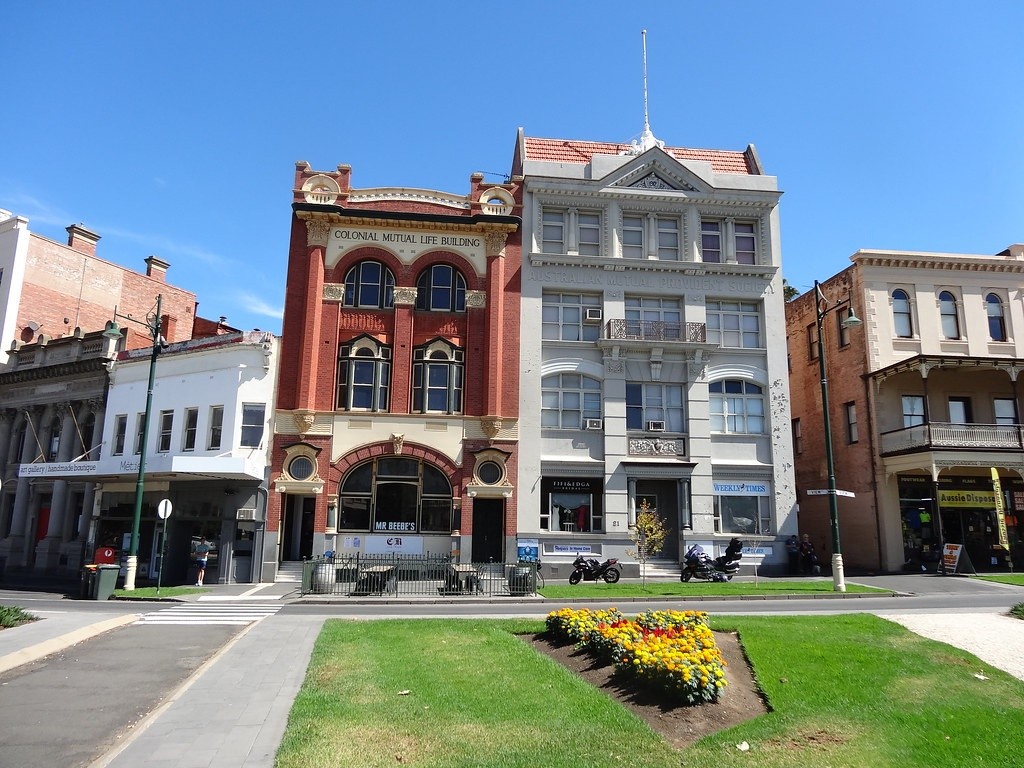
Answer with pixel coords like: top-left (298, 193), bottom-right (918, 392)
top-left (354, 565), bottom-right (398, 597)
top-left (445, 562), bottom-right (487, 596)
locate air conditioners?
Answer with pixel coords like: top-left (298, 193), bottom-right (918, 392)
top-left (236, 508), bottom-right (256, 520)
top-left (584, 308), bottom-right (602, 323)
top-left (583, 418), bottom-right (603, 429)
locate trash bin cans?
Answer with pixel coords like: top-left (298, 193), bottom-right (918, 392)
top-left (80, 564), bottom-right (97, 598)
top-left (93, 563), bottom-right (121, 600)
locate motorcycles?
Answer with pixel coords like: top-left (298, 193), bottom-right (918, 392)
top-left (568, 551), bottom-right (624, 586)
top-left (681, 538), bottom-right (743, 583)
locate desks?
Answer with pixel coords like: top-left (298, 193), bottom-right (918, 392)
top-left (360, 566), bottom-right (394, 595)
top-left (451, 566), bottom-right (478, 595)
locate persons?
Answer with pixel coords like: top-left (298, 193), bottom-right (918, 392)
top-left (785, 535), bottom-right (800, 575)
top-left (194, 536), bottom-right (209, 586)
top-left (800, 534), bottom-right (816, 575)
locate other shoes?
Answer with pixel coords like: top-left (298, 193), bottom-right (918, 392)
top-left (196, 581), bottom-right (203, 586)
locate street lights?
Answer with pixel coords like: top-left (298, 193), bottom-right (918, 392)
top-left (98, 293), bottom-right (162, 593)
top-left (813, 279), bottom-right (862, 593)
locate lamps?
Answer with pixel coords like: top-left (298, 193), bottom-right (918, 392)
top-left (647, 420), bottom-right (666, 432)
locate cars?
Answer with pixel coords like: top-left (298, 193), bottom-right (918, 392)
top-left (190, 536), bottom-right (216, 554)
